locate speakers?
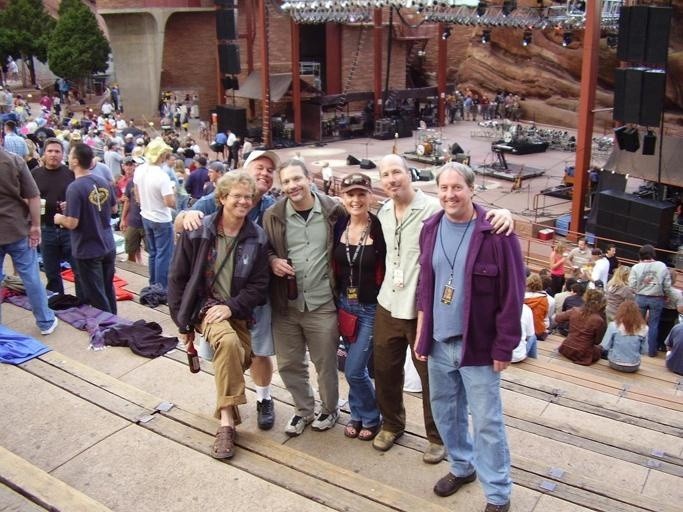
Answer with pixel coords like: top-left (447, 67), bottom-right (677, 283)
top-left (216, 104), bottom-right (246, 146)
top-left (612, 66), bottom-right (667, 127)
top-left (220, 76), bottom-right (238, 90)
top-left (616, 5), bottom-right (672, 65)
top-left (419, 170), bottom-right (434, 182)
top-left (410, 168), bottom-right (420, 182)
top-left (360, 159), bottom-right (376, 169)
top-left (615, 128), bottom-right (656, 156)
top-left (451, 143), bottom-right (464, 156)
top-left (218, 44), bottom-right (240, 74)
top-left (346, 154), bottom-right (360, 165)
top-left (214, 0), bottom-right (239, 6)
top-left (216, 8), bottom-right (235, 40)
top-left (592, 188), bottom-right (676, 266)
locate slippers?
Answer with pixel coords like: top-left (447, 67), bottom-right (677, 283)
top-left (344, 420), bottom-right (361, 437)
top-left (358, 419), bottom-right (382, 441)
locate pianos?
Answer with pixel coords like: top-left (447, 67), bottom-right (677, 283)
top-left (491, 144), bottom-right (514, 152)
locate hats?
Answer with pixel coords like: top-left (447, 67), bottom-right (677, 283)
top-left (340, 173), bottom-right (372, 195)
top-left (72, 131), bottom-right (82, 140)
top-left (243, 149), bottom-right (280, 171)
top-left (34, 117), bottom-right (49, 127)
top-left (25, 122), bottom-right (39, 135)
top-left (123, 133), bottom-right (173, 164)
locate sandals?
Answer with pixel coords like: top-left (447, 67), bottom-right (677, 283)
top-left (211, 426), bottom-right (235, 459)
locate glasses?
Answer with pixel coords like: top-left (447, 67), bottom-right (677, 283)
top-left (228, 194), bottom-right (254, 201)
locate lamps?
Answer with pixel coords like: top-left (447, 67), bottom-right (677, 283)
top-left (289, 6), bottom-right (375, 25)
top-left (614, 124), bottom-right (656, 156)
top-left (424, 5), bottom-right (618, 35)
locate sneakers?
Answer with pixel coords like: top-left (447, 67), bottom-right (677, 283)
top-left (284, 411), bottom-right (314, 437)
top-left (484, 501), bottom-right (510, 511)
top-left (433, 470), bottom-right (477, 497)
top-left (372, 428), bottom-right (404, 451)
top-left (423, 443), bottom-right (445, 463)
top-left (311, 408), bottom-right (340, 431)
top-left (41, 317), bottom-right (57, 335)
top-left (257, 395), bottom-right (274, 429)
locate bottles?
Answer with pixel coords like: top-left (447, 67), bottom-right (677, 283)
top-left (55, 202), bottom-right (61, 232)
top-left (185, 338), bottom-right (202, 374)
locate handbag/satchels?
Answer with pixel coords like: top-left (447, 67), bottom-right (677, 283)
top-left (179, 294), bottom-right (207, 334)
top-left (337, 309), bottom-right (359, 342)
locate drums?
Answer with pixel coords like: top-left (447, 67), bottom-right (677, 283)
top-left (417, 142), bottom-right (432, 155)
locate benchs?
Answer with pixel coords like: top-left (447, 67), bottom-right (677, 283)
top-left (0, 253), bottom-right (682, 512)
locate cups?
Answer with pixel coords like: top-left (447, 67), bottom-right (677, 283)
top-left (39, 199), bottom-right (46, 215)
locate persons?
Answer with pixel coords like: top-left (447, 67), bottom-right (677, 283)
top-left (261, 158), bottom-right (349, 437)
top-left (172, 149), bottom-right (282, 430)
top-left (331, 172), bottom-right (387, 441)
top-left (638, 180), bottom-right (683, 225)
top-left (166, 169), bottom-right (271, 461)
top-left (412, 158), bottom-right (531, 512)
top-left (0, 53), bottom-right (255, 336)
top-left (510, 239), bottom-right (683, 375)
top-left (447, 87), bottom-right (521, 125)
top-left (509, 123), bottom-right (577, 153)
top-left (563, 164), bottom-right (601, 187)
top-left (272, 113), bottom-right (292, 141)
top-left (372, 153), bottom-right (515, 464)
top-left (338, 98), bottom-right (437, 140)
top-left (322, 161), bottom-right (335, 195)
